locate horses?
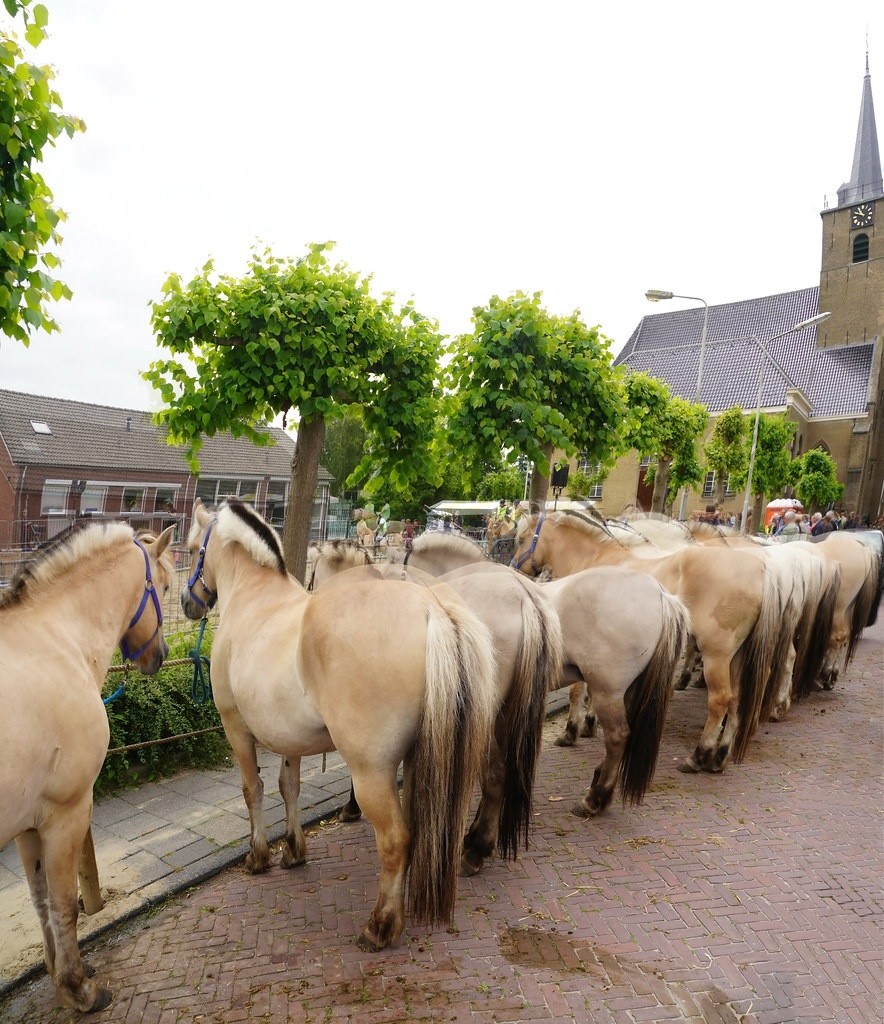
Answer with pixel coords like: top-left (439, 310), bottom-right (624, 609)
top-left (0, 518), bottom-right (180, 1012)
top-left (180, 496), bottom-right (884, 955)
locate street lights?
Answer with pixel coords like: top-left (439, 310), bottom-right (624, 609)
top-left (740, 311), bottom-right (832, 534)
top-left (645, 289), bottom-right (710, 522)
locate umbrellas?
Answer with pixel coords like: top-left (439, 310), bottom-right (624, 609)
top-left (766, 498), bottom-right (804, 508)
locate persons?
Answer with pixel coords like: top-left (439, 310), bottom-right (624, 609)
top-left (377, 512), bottom-right (387, 537)
top-left (781, 512), bottom-right (800, 535)
top-left (699, 505), bottom-right (720, 526)
top-left (727, 507), bottom-right (869, 535)
top-left (486, 499), bottom-right (525, 555)
top-left (402, 511), bottom-right (463, 546)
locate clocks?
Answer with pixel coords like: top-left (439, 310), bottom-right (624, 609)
top-left (851, 199), bottom-right (875, 230)
